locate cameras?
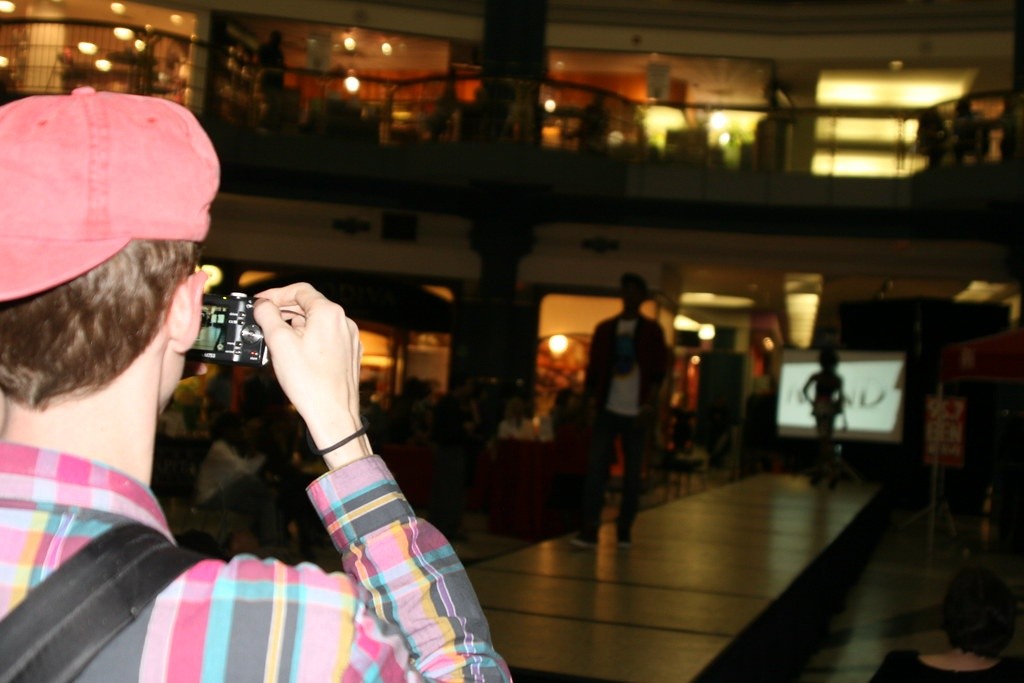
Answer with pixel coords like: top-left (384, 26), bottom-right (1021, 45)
top-left (184, 292), bottom-right (269, 366)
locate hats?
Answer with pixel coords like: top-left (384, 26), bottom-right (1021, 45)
top-left (0, 85), bottom-right (221, 303)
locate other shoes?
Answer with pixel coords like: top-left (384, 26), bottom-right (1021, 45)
top-left (568, 526), bottom-right (599, 549)
top-left (616, 527), bottom-right (632, 548)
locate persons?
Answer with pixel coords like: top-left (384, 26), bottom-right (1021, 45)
top-left (461, 68), bottom-right (515, 142)
top-left (256, 31), bottom-right (286, 130)
top-left (0, 86), bottom-right (511, 683)
top-left (166, 351), bottom-right (598, 562)
top-left (566, 92), bottom-right (610, 155)
top-left (567, 272), bottom-right (670, 551)
top-left (916, 99), bottom-right (988, 167)
top-left (869, 567), bottom-right (1024, 683)
top-left (804, 348), bottom-right (844, 489)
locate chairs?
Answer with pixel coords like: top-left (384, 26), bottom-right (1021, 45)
top-left (379, 441), bottom-right (437, 513)
top-left (478, 422), bottom-right (591, 543)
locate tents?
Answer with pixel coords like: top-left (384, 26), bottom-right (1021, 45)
top-left (925, 328), bottom-right (1024, 554)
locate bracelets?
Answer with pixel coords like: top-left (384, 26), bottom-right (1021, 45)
top-left (306, 416), bottom-right (369, 455)
